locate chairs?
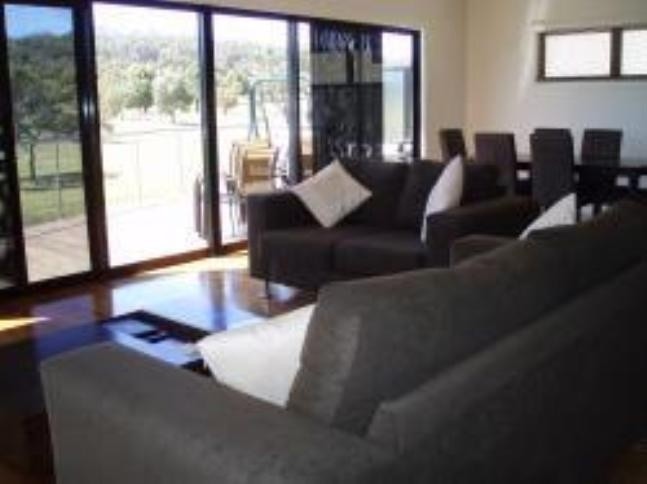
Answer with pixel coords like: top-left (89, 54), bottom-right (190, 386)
top-left (36, 157), bottom-right (646, 484)
top-left (438, 128), bottom-right (622, 212)
top-left (195, 137), bottom-right (314, 241)
